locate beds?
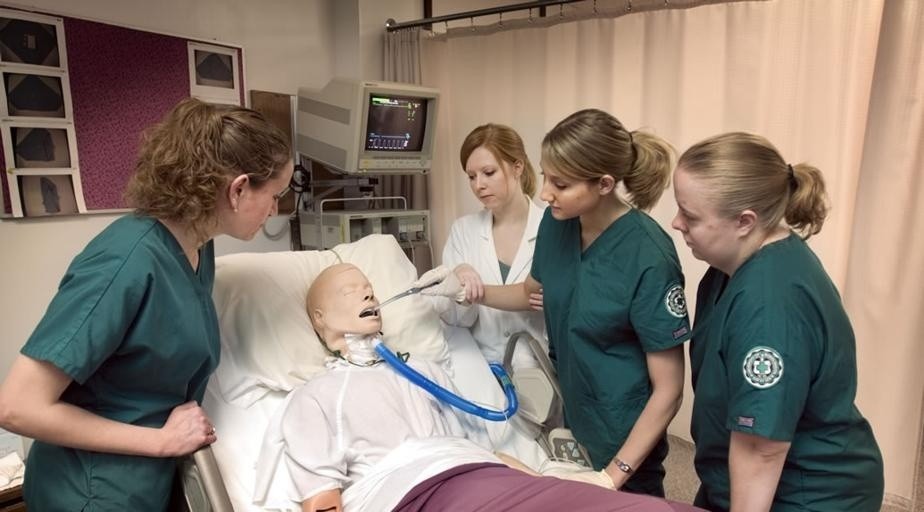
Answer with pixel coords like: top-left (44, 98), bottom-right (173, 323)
top-left (179, 327), bottom-right (597, 512)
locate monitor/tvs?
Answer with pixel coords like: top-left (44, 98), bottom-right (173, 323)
top-left (296, 77), bottom-right (442, 177)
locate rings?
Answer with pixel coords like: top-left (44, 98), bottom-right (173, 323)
top-left (210, 425), bottom-right (216, 434)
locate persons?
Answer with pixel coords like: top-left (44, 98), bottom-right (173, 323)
top-left (412, 109), bottom-right (696, 498)
top-left (280, 263), bottom-right (704, 512)
top-left (673, 131), bottom-right (884, 511)
top-left (0, 97), bottom-right (294, 511)
top-left (442, 122), bottom-right (550, 370)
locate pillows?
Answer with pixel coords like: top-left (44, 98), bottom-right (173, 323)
top-left (203, 231), bottom-right (454, 383)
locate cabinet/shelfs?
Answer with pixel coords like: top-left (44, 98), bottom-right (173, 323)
top-left (303, 210), bottom-right (433, 250)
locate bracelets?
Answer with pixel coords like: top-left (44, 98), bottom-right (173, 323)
top-left (612, 456), bottom-right (633, 474)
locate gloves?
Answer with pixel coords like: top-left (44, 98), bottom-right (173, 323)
top-left (411, 265), bottom-right (467, 302)
top-left (539, 461), bottom-right (618, 489)
